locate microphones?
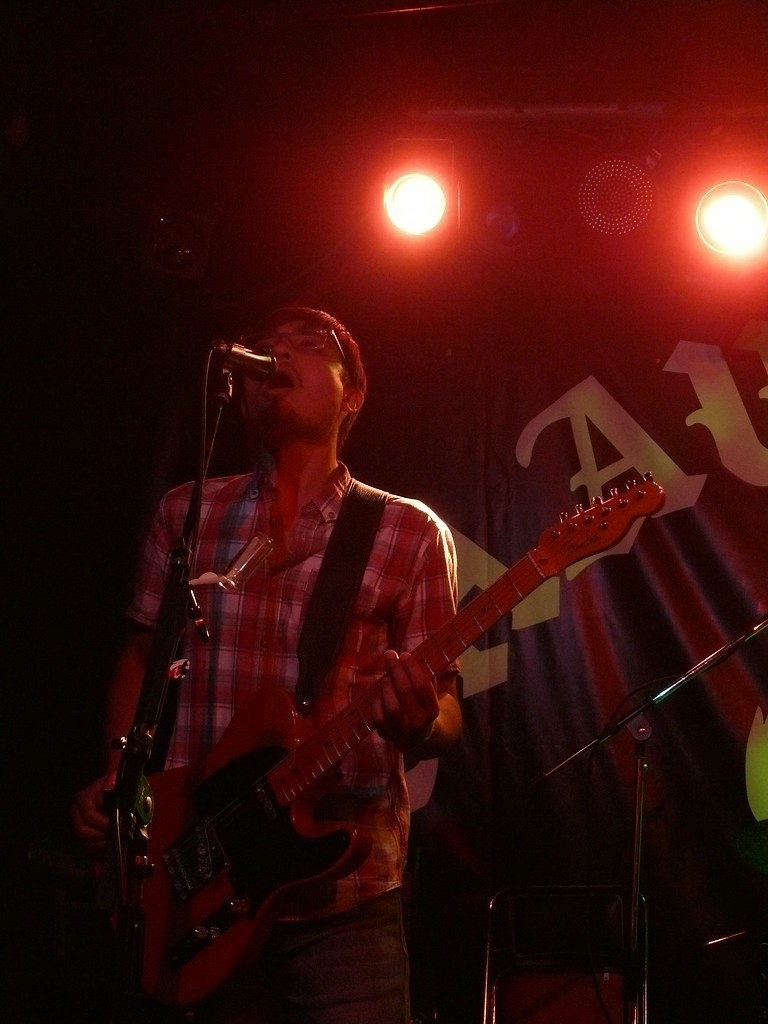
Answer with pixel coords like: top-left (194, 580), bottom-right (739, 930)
top-left (214, 345), bottom-right (278, 380)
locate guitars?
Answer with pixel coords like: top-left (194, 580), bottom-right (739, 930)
top-left (92, 472), bottom-right (667, 1014)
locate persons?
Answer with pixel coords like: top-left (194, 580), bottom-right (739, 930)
top-left (71, 307), bottom-right (461, 1024)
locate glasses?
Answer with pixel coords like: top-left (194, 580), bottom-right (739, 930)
top-left (244, 329), bottom-right (346, 360)
